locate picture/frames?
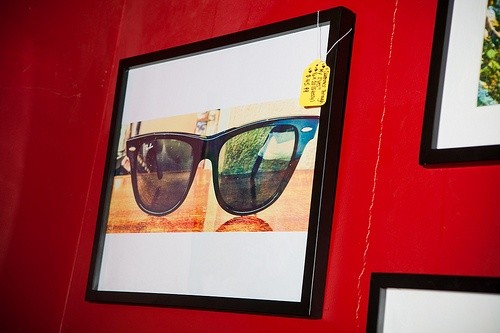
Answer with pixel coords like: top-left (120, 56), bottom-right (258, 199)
top-left (85, 4), bottom-right (357, 319)
top-left (418, 0), bottom-right (500, 167)
top-left (364, 272), bottom-right (500, 333)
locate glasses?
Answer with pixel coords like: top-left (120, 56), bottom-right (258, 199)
top-left (125, 116), bottom-right (320, 216)
